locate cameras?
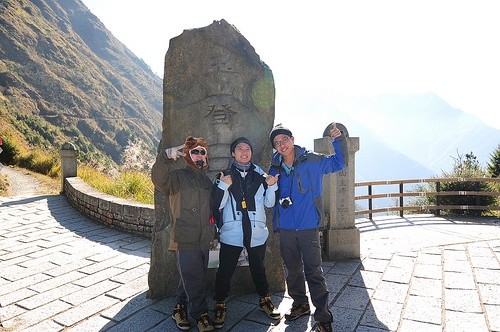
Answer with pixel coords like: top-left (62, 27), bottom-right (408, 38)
top-left (279, 197), bottom-right (292, 209)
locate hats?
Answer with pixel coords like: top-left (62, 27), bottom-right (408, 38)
top-left (269, 123), bottom-right (293, 149)
top-left (230, 136), bottom-right (253, 158)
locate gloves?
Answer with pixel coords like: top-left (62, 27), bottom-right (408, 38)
top-left (164, 144), bottom-right (188, 162)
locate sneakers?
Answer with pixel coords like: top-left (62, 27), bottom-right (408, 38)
top-left (313, 321), bottom-right (333, 332)
top-left (171, 302), bottom-right (191, 330)
top-left (213, 302), bottom-right (227, 329)
top-left (193, 312), bottom-right (216, 332)
top-left (284, 303), bottom-right (311, 321)
top-left (259, 295), bottom-right (281, 320)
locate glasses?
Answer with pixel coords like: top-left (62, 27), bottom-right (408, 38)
top-left (274, 137), bottom-right (291, 147)
top-left (191, 149), bottom-right (205, 155)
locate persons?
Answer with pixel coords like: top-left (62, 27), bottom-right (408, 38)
top-left (211, 137), bottom-right (280, 329)
top-left (151, 137), bottom-right (219, 332)
top-left (265, 122), bottom-right (349, 332)
